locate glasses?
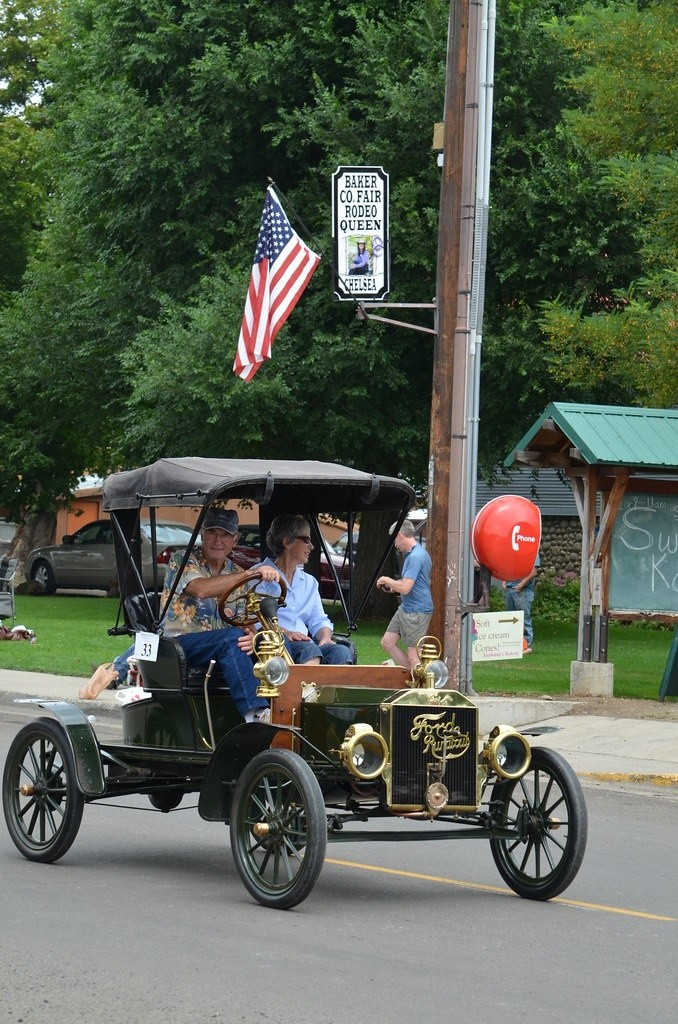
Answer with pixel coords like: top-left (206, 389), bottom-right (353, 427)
top-left (289, 536), bottom-right (312, 543)
top-left (201, 529), bottom-right (238, 540)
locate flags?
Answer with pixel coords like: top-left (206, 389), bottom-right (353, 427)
top-left (232, 186), bottom-right (322, 382)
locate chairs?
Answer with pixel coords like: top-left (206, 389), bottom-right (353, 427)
top-left (0, 557), bottom-right (19, 628)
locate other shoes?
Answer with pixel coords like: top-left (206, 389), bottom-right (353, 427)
top-left (92, 662), bottom-right (118, 690)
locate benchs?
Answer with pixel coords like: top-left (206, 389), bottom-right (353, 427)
top-left (124, 591), bottom-right (350, 695)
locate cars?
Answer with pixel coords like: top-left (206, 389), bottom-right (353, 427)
top-left (232, 524), bottom-right (355, 608)
top-left (24, 517), bottom-right (233, 597)
top-left (329, 528), bottom-right (427, 563)
top-left (3, 453), bottom-right (587, 912)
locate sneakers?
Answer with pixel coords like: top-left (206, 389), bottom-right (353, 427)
top-left (253, 707), bottom-right (270, 722)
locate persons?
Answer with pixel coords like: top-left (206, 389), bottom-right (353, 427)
top-left (244, 513), bottom-right (353, 665)
top-left (158, 506), bottom-right (273, 724)
top-left (376, 519), bottom-right (435, 687)
top-left (474, 549), bottom-right (541, 653)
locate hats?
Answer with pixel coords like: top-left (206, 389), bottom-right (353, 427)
top-left (202, 507), bottom-right (239, 535)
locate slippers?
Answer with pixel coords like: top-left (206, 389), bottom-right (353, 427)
top-left (523, 648), bottom-right (532, 655)
